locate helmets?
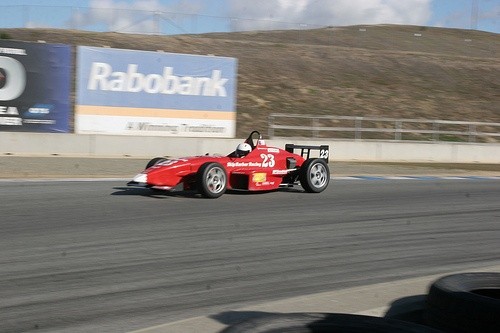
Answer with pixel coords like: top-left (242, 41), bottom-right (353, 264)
top-left (236, 143), bottom-right (252, 152)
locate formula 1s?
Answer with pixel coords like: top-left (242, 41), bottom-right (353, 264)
top-left (114, 129), bottom-right (330, 199)
